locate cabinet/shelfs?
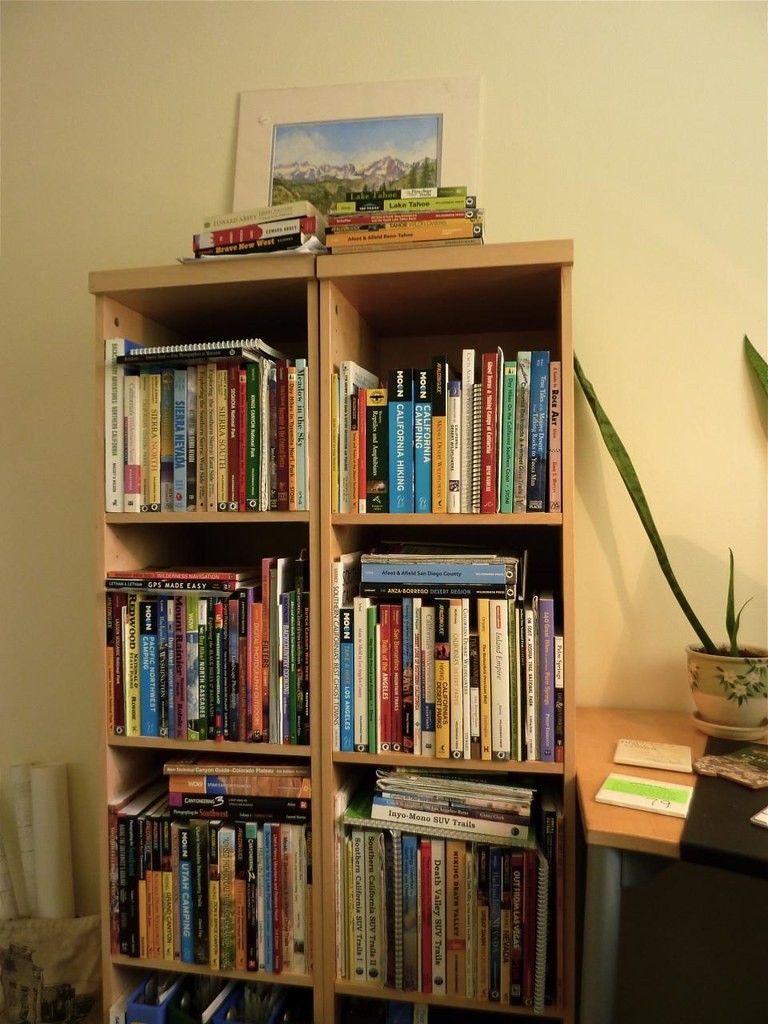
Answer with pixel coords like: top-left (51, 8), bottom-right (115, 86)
top-left (87, 255), bottom-right (323, 1023)
top-left (316, 229), bottom-right (574, 1024)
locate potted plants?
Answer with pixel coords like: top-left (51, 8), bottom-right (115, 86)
top-left (574, 352), bottom-right (767, 738)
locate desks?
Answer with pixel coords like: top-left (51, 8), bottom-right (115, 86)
top-left (572, 709), bottom-right (768, 1024)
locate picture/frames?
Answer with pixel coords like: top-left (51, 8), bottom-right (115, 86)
top-left (232, 80), bottom-right (480, 213)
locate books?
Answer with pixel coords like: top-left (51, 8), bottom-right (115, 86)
top-left (325, 341), bottom-right (570, 1023)
top-left (175, 179), bottom-right (487, 264)
top-left (93, 328), bottom-right (316, 1024)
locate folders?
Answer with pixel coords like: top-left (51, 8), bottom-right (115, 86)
top-left (126, 970), bottom-right (292, 1024)
